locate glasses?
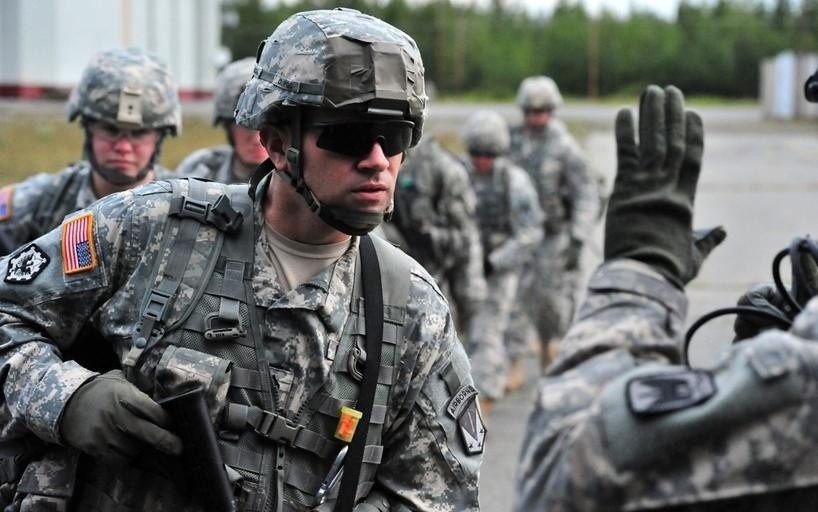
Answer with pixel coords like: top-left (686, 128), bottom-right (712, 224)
top-left (309, 122), bottom-right (406, 157)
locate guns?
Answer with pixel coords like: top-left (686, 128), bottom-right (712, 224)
top-left (136, 385), bottom-right (238, 512)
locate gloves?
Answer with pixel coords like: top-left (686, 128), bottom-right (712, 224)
top-left (59, 365), bottom-right (183, 484)
top-left (605, 85), bottom-right (726, 292)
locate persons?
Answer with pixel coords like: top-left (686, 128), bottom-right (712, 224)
top-left (175, 55), bottom-right (270, 183)
top-left (514, 68), bottom-right (818, 512)
top-left (1, 6), bottom-right (487, 512)
top-left (459, 107), bottom-right (546, 392)
top-left (1, 46), bottom-right (182, 257)
top-left (384, 131), bottom-right (508, 418)
top-left (508, 75), bottom-right (602, 376)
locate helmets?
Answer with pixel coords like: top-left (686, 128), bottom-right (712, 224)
top-left (464, 113), bottom-right (512, 150)
top-left (68, 45), bottom-right (182, 137)
top-left (514, 76), bottom-right (561, 112)
top-left (211, 57), bottom-right (257, 126)
top-left (233, 8), bottom-right (429, 148)
